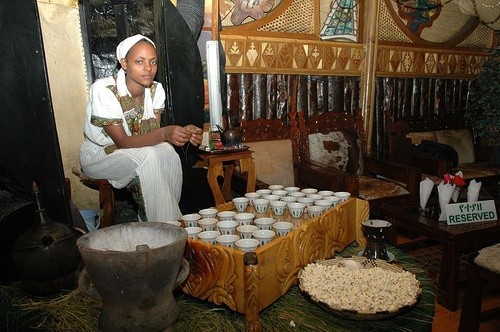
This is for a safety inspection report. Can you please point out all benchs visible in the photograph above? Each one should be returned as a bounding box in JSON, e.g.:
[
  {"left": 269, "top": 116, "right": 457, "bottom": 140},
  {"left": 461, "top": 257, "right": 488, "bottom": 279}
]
[{"left": 384, "top": 108, "right": 500, "bottom": 196}]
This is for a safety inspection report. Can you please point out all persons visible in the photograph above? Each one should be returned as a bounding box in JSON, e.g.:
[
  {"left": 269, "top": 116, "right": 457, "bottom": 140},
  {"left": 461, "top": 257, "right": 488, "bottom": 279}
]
[{"left": 80, "top": 35, "right": 202, "bottom": 227}]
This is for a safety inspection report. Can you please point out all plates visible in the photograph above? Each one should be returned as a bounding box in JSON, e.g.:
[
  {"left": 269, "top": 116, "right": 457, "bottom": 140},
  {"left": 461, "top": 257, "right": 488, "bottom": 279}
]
[{"left": 357, "top": 250, "right": 396, "bottom": 263}]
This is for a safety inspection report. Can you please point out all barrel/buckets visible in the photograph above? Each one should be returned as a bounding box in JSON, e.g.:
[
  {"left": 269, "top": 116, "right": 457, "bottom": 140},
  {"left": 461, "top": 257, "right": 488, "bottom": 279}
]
[{"left": 79, "top": 209, "right": 97, "bottom": 232}]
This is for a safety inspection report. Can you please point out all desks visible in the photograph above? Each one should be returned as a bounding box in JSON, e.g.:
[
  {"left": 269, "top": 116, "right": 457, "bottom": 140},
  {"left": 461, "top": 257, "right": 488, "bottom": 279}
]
[
  {"left": 0, "top": 239, "right": 435, "bottom": 332},
  {"left": 197, "top": 151, "right": 258, "bottom": 206},
  {"left": 381, "top": 199, "right": 500, "bottom": 311}
]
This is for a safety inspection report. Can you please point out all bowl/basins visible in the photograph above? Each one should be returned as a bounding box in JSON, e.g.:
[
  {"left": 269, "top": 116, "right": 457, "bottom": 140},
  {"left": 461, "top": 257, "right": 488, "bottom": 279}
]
[
  {"left": 231, "top": 184, "right": 351, "bottom": 219},
  {"left": 165, "top": 207, "right": 293, "bottom": 251}
]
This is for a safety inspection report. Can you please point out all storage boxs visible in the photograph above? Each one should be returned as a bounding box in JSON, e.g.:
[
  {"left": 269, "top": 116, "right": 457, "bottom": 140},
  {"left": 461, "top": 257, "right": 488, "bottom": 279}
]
[{"left": 179, "top": 184, "right": 356, "bottom": 332}]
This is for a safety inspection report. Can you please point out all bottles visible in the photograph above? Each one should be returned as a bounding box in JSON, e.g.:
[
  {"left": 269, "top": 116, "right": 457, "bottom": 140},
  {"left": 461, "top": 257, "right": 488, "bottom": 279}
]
[{"left": 361, "top": 219, "right": 392, "bottom": 261}]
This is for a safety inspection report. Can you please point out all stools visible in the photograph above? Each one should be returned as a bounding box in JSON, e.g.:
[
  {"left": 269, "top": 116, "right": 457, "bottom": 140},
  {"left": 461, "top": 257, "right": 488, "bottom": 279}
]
[{"left": 72, "top": 166, "right": 120, "bottom": 227}]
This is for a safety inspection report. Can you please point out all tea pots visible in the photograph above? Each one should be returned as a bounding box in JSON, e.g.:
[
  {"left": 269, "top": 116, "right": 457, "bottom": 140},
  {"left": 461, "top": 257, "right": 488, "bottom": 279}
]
[{"left": 216, "top": 118, "right": 246, "bottom": 146}]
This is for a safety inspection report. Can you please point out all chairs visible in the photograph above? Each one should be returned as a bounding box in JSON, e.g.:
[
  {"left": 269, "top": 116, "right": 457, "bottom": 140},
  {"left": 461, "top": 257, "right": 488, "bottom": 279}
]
[
  {"left": 230, "top": 114, "right": 343, "bottom": 199},
  {"left": 298, "top": 107, "right": 421, "bottom": 219},
  {"left": 458, "top": 242, "right": 500, "bottom": 332}
]
